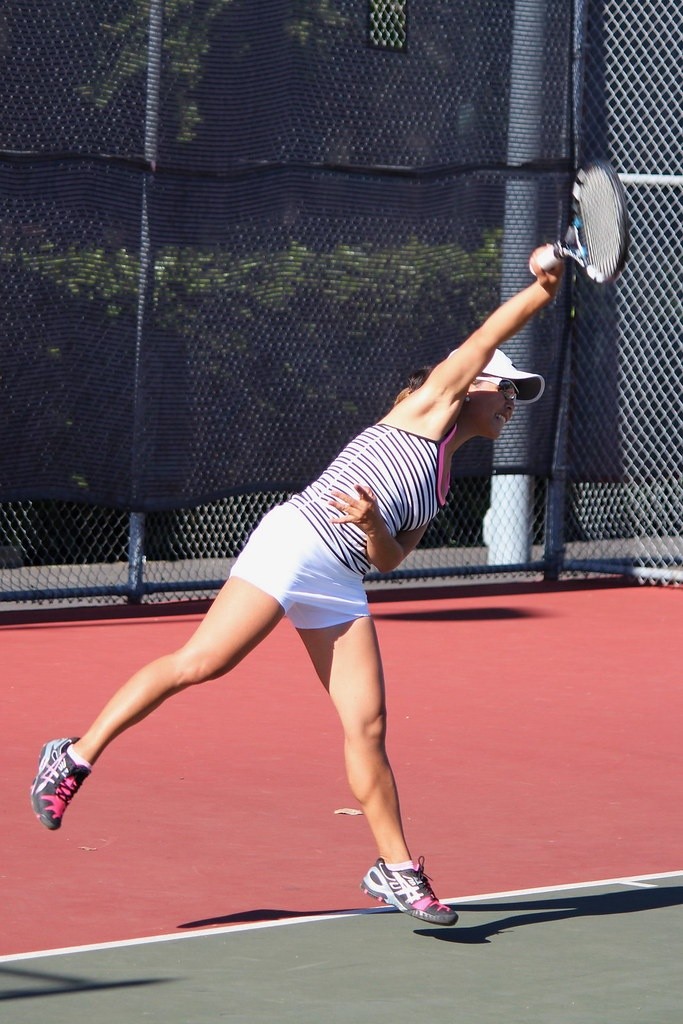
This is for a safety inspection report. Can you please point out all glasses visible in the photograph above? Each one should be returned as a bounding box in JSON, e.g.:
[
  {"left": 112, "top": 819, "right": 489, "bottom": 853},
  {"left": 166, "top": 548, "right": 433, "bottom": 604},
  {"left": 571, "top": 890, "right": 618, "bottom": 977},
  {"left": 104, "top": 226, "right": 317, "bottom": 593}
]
[{"left": 476, "top": 376, "right": 519, "bottom": 405}]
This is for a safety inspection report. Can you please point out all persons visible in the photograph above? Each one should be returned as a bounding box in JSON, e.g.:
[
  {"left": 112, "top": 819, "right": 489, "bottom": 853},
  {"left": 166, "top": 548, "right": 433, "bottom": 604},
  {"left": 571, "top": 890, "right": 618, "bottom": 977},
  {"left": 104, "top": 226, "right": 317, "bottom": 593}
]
[{"left": 29, "top": 242, "right": 563, "bottom": 926}]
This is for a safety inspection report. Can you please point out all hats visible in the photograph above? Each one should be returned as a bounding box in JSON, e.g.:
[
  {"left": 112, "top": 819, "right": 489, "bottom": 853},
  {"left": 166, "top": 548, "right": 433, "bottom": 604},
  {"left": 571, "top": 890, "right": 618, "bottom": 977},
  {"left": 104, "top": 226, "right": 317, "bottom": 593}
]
[{"left": 482, "top": 349, "right": 545, "bottom": 405}]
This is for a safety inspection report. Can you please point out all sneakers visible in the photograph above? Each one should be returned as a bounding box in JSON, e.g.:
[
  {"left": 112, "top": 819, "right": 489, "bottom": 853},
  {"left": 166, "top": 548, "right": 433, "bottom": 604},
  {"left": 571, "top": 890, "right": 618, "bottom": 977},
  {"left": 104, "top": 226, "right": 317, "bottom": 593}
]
[
  {"left": 30, "top": 737, "right": 91, "bottom": 830},
  {"left": 360, "top": 856, "right": 459, "bottom": 925}
]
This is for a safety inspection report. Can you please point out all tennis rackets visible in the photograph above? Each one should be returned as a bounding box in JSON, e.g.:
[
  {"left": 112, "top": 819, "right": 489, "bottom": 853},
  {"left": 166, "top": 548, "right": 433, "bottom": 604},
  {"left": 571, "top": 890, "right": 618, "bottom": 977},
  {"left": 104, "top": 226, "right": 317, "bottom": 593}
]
[{"left": 531, "top": 158, "right": 629, "bottom": 286}]
